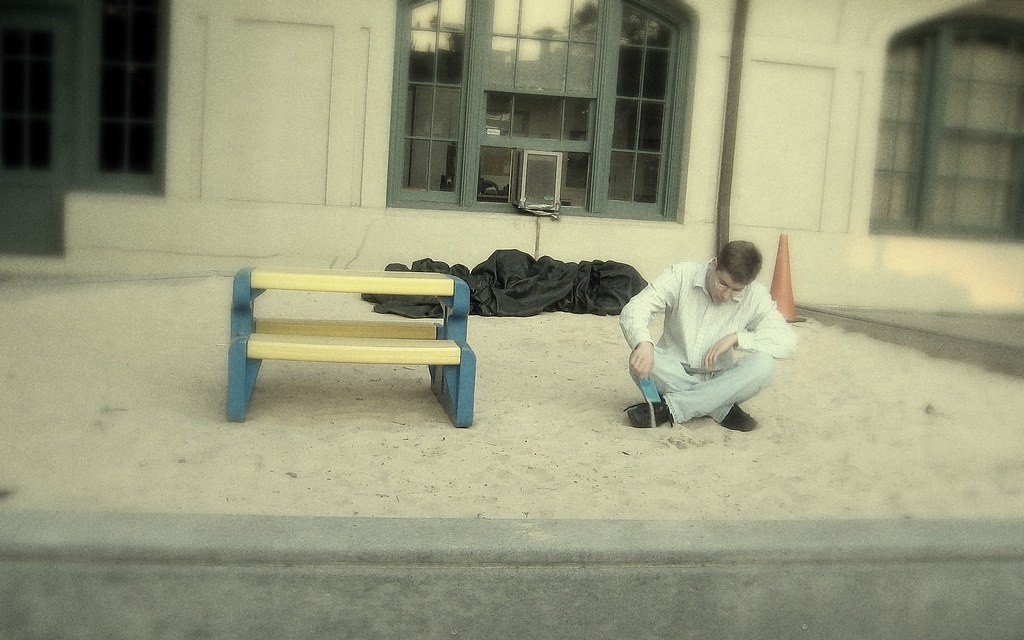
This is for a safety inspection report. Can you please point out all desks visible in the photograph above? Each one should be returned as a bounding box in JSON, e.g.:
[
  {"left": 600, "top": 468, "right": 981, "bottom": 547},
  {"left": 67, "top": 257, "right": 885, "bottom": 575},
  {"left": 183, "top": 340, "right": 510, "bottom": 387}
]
[{"left": 231, "top": 265, "right": 470, "bottom": 342}]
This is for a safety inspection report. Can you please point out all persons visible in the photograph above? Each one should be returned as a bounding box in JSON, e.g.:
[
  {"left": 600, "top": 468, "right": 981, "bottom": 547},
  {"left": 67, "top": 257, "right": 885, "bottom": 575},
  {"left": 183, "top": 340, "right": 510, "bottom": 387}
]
[{"left": 619, "top": 241, "right": 796, "bottom": 431}]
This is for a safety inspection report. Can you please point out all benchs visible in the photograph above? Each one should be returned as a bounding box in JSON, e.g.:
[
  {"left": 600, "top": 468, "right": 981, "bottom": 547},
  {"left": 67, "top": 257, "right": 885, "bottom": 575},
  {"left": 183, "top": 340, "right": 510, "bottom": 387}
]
[{"left": 226, "top": 321, "right": 477, "bottom": 428}]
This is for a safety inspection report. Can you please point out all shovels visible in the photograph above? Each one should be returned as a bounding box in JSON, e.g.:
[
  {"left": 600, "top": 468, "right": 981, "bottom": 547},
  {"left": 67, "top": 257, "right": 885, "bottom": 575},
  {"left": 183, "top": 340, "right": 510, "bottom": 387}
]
[{"left": 630, "top": 372, "right": 662, "bottom": 403}]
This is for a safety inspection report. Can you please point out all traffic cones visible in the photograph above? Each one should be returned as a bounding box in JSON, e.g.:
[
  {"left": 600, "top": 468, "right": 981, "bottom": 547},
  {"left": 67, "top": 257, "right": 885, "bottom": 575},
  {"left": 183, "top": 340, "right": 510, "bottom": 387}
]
[{"left": 770, "top": 234, "right": 808, "bottom": 323}]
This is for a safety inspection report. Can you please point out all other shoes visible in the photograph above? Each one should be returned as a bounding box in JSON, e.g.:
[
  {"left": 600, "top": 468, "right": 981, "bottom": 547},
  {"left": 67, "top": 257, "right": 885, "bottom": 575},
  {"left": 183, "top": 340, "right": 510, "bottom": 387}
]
[
  {"left": 719, "top": 403, "right": 758, "bottom": 432},
  {"left": 623, "top": 396, "right": 674, "bottom": 428}
]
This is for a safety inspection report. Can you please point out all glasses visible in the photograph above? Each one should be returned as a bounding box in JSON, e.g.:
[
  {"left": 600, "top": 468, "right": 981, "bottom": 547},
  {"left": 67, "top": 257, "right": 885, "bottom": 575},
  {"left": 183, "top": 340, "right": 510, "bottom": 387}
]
[{"left": 714, "top": 269, "right": 749, "bottom": 302}]
